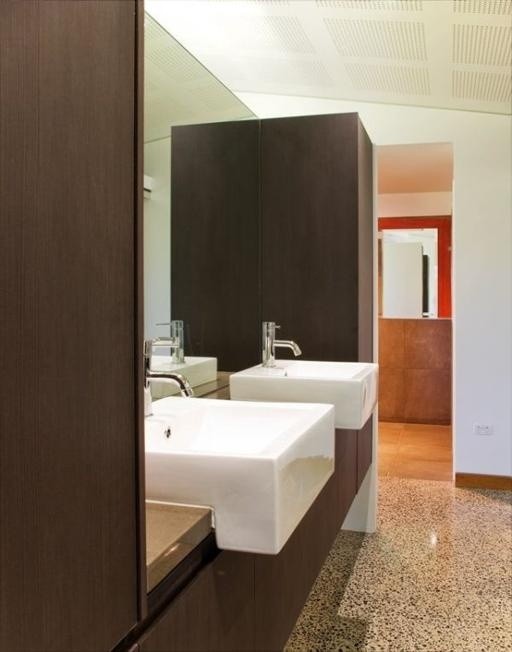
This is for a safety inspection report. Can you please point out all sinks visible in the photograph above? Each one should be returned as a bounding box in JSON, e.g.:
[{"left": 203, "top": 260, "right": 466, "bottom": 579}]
[
  {"left": 150, "top": 355, "right": 218, "bottom": 388},
  {"left": 144, "top": 395, "right": 336, "bottom": 556},
  {"left": 229, "top": 359, "right": 379, "bottom": 430}
]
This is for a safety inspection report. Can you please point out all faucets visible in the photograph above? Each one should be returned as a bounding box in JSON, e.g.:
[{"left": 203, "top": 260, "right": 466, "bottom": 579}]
[
  {"left": 261, "top": 322, "right": 302, "bottom": 368},
  {"left": 143, "top": 340, "right": 195, "bottom": 418},
  {"left": 150, "top": 320, "right": 184, "bottom": 364}
]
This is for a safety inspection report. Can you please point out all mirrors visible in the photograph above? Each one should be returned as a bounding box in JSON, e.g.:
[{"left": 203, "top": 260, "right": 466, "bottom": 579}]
[{"left": 140, "top": 10, "right": 262, "bottom": 420}]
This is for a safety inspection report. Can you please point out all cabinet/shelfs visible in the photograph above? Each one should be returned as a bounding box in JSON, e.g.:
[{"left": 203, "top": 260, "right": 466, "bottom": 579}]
[{"left": 77, "top": 414, "right": 377, "bottom": 651}]
